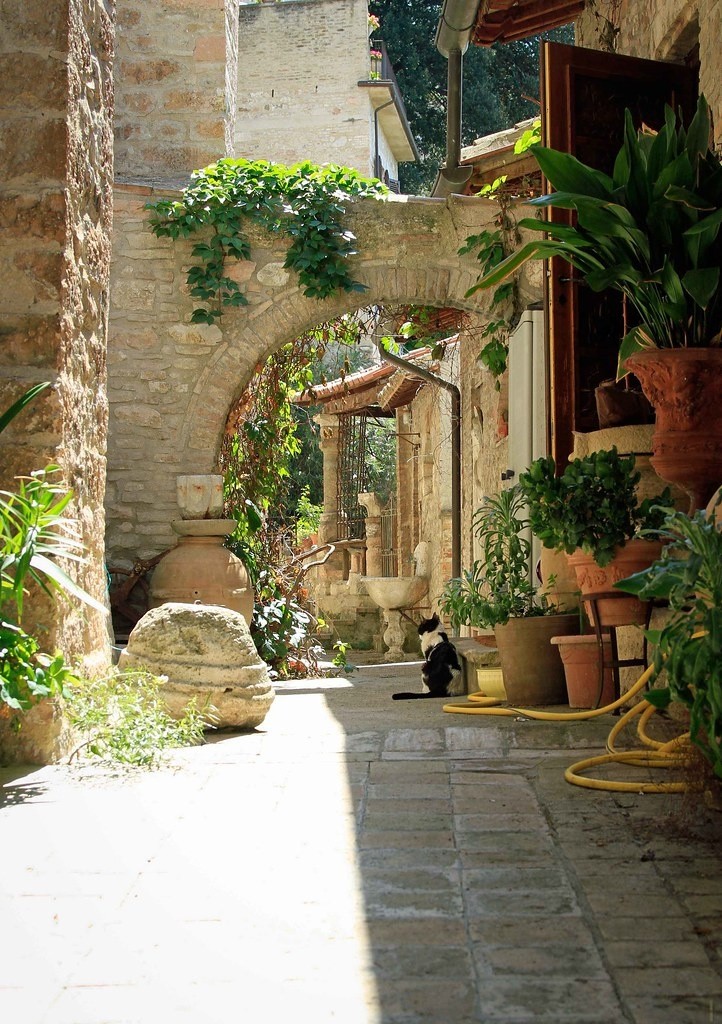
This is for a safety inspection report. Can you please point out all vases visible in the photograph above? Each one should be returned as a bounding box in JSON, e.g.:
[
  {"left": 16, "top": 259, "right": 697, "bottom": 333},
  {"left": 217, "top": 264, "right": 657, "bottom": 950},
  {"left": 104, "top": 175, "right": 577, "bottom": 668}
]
[
  {"left": 367, "top": 25, "right": 374, "bottom": 37},
  {"left": 371, "top": 60, "right": 381, "bottom": 72},
  {"left": 475, "top": 667, "right": 507, "bottom": 701},
  {"left": 550, "top": 634, "right": 616, "bottom": 708}
]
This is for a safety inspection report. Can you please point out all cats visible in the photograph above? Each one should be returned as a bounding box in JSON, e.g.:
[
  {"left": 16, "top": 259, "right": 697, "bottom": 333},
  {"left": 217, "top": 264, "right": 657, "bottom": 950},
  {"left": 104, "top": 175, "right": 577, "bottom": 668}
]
[{"left": 392, "top": 613, "right": 463, "bottom": 701}]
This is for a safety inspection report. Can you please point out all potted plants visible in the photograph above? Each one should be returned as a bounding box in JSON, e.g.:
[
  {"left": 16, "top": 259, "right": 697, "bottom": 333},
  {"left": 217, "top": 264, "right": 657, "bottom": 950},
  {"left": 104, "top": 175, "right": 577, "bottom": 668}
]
[
  {"left": 519, "top": 445, "right": 675, "bottom": 627},
  {"left": 432, "top": 482, "right": 580, "bottom": 704},
  {"left": 296, "top": 485, "right": 323, "bottom": 552},
  {"left": 464, "top": 86, "right": 721, "bottom": 522}
]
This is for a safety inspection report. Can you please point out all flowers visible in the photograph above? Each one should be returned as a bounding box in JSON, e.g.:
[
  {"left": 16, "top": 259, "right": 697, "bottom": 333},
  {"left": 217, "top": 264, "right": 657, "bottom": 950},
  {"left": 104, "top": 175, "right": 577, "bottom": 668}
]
[
  {"left": 368, "top": 13, "right": 381, "bottom": 31},
  {"left": 370, "top": 50, "right": 383, "bottom": 61}
]
